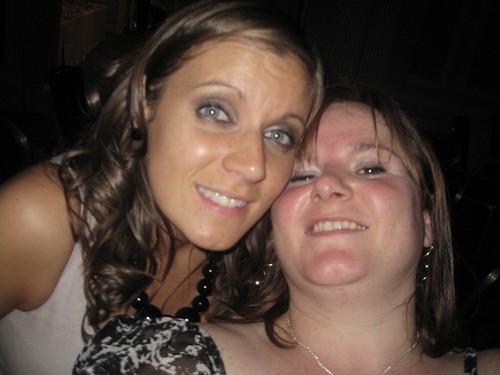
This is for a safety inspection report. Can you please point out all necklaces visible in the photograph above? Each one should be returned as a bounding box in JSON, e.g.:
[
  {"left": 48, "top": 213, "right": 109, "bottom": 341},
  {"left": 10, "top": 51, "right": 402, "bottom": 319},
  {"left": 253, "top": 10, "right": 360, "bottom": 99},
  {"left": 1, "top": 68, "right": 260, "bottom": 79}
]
[
  {"left": 286, "top": 310, "right": 421, "bottom": 375},
  {"left": 127, "top": 244, "right": 221, "bottom": 323}
]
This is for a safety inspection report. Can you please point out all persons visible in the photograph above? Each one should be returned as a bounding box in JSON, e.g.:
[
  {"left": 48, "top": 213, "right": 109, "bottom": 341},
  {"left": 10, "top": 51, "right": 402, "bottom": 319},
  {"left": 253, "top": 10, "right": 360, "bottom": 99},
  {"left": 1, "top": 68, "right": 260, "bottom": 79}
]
[
  {"left": 0, "top": 0, "right": 328, "bottom": 375},
  {"left": 69, "top": 79, "right": 500, "bottom": 375}
]
